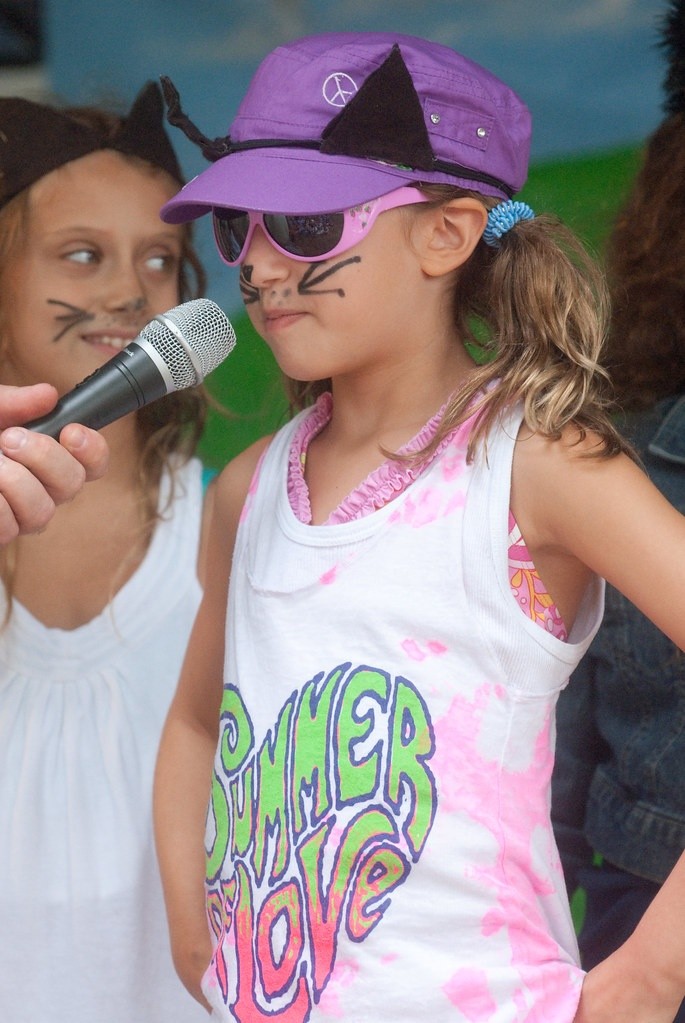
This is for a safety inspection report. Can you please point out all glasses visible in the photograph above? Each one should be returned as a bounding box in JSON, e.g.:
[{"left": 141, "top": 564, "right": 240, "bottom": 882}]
[{"left": 212, "top": 187, "right": 457, "bottom": 267}]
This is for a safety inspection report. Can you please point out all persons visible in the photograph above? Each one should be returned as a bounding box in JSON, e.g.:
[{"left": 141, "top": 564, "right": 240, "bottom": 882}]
[
  {"left": 0, "top": 80, "right": 217, "bottom": 1023},
  {"left": 0, "top": 381, "right": 111, "bottom": 548},
  {"left": 554, "top": 1, "right": 685, "bottom": 1023},
  {"left": 151, "top": 35, "right": 684, "bottom": 1023}
]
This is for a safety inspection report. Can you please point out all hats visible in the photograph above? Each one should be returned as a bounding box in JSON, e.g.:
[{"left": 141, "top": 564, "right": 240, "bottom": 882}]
[{"left": 159, "top": 29, "right": 532, "bottom": 224}]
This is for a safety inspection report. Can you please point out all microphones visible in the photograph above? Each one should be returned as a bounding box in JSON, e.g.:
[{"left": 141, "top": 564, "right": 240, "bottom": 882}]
[{"left": 20, "top": 298, "right": 238, "bottom": 444}]
